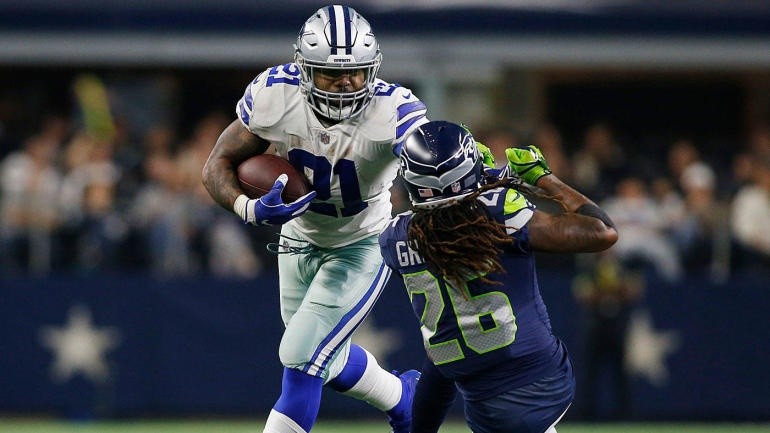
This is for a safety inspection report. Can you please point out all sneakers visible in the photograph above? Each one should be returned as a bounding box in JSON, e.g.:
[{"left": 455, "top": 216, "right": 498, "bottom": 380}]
[{"left": 386, "top": 369, "right": 421, "bottom": 433}]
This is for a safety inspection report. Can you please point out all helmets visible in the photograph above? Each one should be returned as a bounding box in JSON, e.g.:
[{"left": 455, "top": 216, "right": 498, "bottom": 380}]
[
  {"left": 401, "top": 122, "right": 480, "bottom": 210},
  {"left": 293, "top": 4, "right": 383, "bottom": 121}
]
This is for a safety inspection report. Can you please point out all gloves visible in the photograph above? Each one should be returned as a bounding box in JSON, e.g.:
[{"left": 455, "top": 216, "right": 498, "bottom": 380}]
[
  {"left": 233, "top": 173, "right": 316, "bottom": 227},
  {"left": 475, "top": 142, "right": 495, "bottom": 169},
  {"left": 506, "top": 145, "right": 552, "bottom": 186}
]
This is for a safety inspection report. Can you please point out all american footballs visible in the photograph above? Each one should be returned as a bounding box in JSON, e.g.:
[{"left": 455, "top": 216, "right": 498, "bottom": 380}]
[{"left": 235, "top": 154, "right": 307, "bottom": 204}]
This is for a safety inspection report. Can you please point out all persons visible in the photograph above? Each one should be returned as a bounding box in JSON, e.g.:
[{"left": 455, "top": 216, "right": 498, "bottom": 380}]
[
  {"left": 0, "top": 113, "right": 263, "bottom": 287},
  {"left": 378, "top": 120, "right": 620, "bottom": 433},
  {"left": 201, "top": 5, "right": 493, "bottom": 433},
  {"left": 491, "top": 120, "right": 770, "bottom": 279}
]
